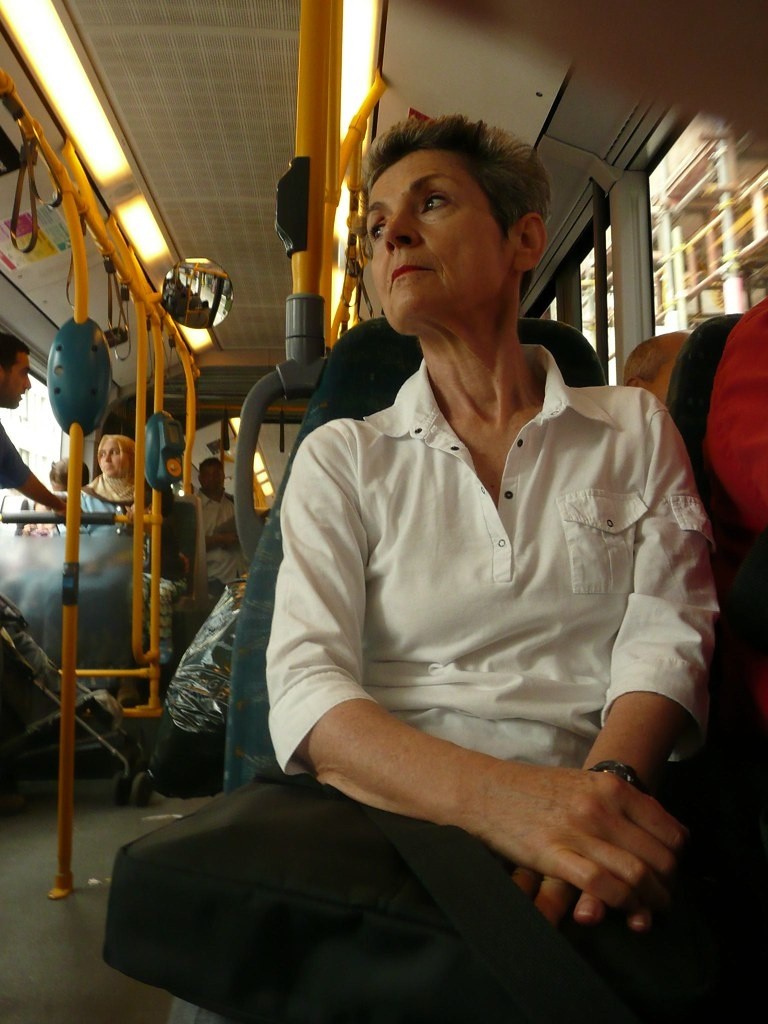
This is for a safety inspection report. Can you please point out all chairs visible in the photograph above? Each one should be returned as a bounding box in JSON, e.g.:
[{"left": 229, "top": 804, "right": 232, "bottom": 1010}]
[
  {"left": 166, "top": 495, "right": 209, "bottom": 652},
  {"left": 226, "top": 316, "right": 601, "bottom": 797},
  {"left": 668, "top": 315, "right": 768, "bottom": 701},
  {"left": 0, "top": 492, "right": 68, "bottom": 535}
]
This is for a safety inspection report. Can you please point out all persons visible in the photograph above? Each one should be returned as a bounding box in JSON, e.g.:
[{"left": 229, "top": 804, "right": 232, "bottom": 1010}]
[
  {"left": 23, "top": 413, "right": 191, "bottom": 708},
  {"left": 0, "top": 332, "right": 84, "bottom": 517},
  {"left": 705, "top": 295, "right": 768, "bottom": 732},
  {"left": 192, "top": 457, "right": 243, "bottom": 595},
  {"left": 166, "top": 116, "right": 721, "bottom": 1023},
  {"left": 623, "top": 331, "right": 688, "bottom": 403}
]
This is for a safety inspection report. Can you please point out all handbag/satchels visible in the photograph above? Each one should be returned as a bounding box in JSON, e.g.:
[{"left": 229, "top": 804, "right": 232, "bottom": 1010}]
[
  {"left": 146, "top": 572, "right": 247, "bottom": 799},
  {"left": 103, "top": 775, "right": 724, "bottom": 1024}
]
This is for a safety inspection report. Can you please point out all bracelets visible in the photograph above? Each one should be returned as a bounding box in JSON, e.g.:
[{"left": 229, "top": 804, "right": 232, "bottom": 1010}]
[{"left": 585, "top": 760, "right": 649, "bottom": 795}]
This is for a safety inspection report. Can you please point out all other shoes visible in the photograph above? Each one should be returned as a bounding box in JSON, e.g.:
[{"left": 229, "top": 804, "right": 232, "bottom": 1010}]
[{"left": 0, "top": 793, "right": 25, "bottom": 816}]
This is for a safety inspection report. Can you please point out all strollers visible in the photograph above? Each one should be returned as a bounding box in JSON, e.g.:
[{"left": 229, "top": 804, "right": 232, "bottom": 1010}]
[{"left": 0, "top": 593, "right": 154, "bottom": 806}]
